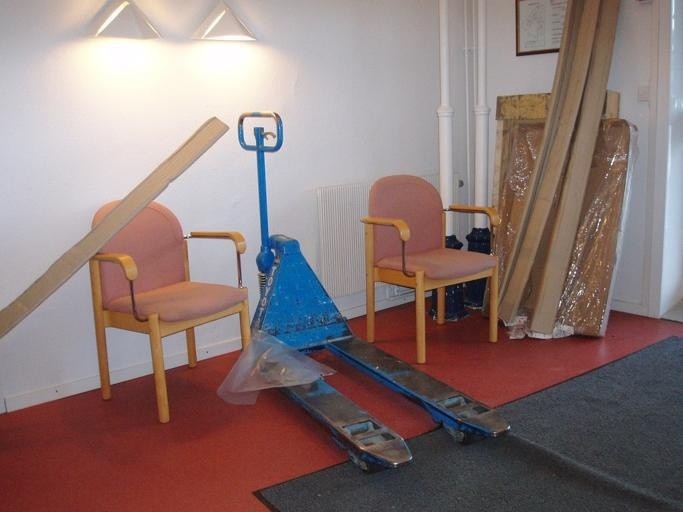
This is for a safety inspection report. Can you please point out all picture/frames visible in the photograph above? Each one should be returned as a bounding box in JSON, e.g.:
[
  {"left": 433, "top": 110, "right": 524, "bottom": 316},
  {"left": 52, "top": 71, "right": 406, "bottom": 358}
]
[{"left": 515, "top": 0, "right": 568, "bottom": 58}]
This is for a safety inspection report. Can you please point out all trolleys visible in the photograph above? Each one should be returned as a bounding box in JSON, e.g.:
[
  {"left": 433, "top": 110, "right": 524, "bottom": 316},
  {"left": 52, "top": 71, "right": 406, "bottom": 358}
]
[{"left": 238, "top": 112, "right": 510, "bottom": 472}]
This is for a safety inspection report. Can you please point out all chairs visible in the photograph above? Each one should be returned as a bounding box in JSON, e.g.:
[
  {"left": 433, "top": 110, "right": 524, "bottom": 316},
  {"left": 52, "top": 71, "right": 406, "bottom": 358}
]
[
  {"left": 363, "top": 173, "right": 500, "bottom": 365},
  {"left": 89, "top": 201, "right": 251, "bottom": 426}
]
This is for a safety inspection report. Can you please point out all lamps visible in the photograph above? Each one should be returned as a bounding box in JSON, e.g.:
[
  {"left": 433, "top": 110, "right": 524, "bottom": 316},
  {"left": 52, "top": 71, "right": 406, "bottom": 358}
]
[
  {"left": 95, "top": 0, "right": 161, "bottom": 41},
  {"left": 191, "top": 0, "right": 254, "bottom": 41}
]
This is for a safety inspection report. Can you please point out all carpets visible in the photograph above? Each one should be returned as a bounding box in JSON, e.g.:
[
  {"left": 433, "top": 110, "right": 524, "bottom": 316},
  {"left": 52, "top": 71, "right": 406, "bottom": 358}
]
[{"left": 249, "top": 334, "right": 682, "bottom": 512}]
